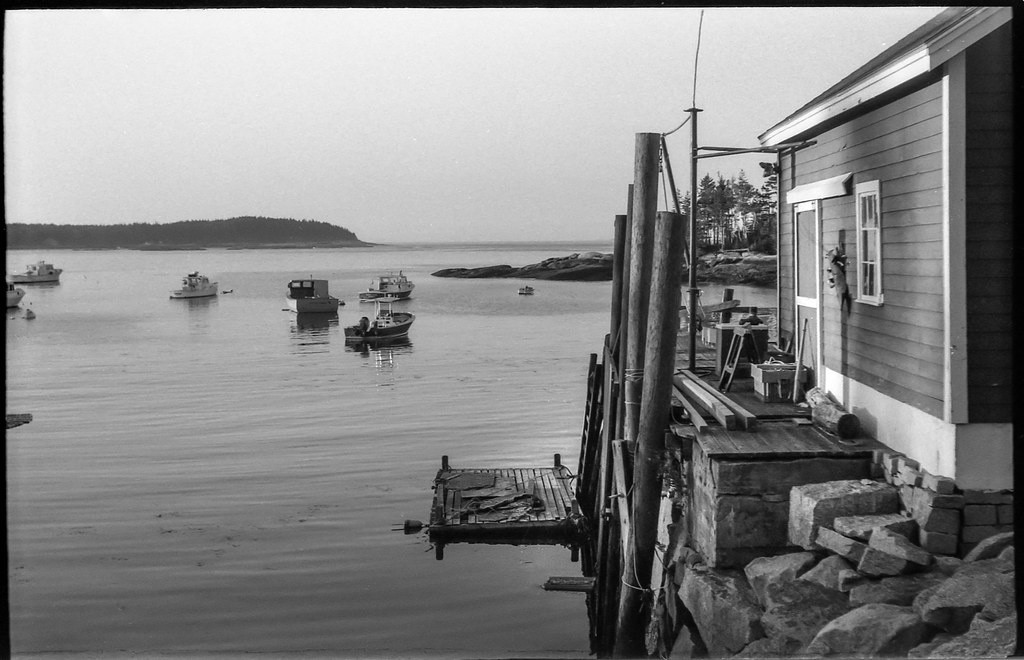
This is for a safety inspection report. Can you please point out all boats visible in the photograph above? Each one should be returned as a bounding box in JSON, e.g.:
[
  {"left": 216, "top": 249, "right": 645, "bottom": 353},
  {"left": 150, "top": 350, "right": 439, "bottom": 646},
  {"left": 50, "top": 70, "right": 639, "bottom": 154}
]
[
  {"left": 359, "top": 270, "right": 415, "bottom": 300},
  {"left": 169, "top": 271, "right": 218, "bottom": 297},
  {"left": 285, "top": 279, "right": 339, "bottom": 313},
  {"left": 344, "top": 296, "right": 415, "bottom": 339},
  {"left": 6, "top": 279, "right": 25, "bottom": 306},
  {"left": 518, "top": 285, "right": 534, "bottom": 295},
  {"left": 8, "top": 260, "right": 63, "bottom": 283}
]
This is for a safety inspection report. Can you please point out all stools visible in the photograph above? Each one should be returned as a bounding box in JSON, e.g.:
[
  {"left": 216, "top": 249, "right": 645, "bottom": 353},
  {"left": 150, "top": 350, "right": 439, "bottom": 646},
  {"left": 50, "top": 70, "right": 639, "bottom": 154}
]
[{"left": 715, "top": 323, "right": 769, "bottom": 378}]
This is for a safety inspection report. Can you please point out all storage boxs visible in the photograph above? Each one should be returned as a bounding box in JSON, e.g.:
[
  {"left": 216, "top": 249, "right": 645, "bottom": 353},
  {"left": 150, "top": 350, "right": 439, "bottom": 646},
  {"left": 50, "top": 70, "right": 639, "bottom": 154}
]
[{"left": 749, "top": 363, "right": 807, "bottom": 404}]
